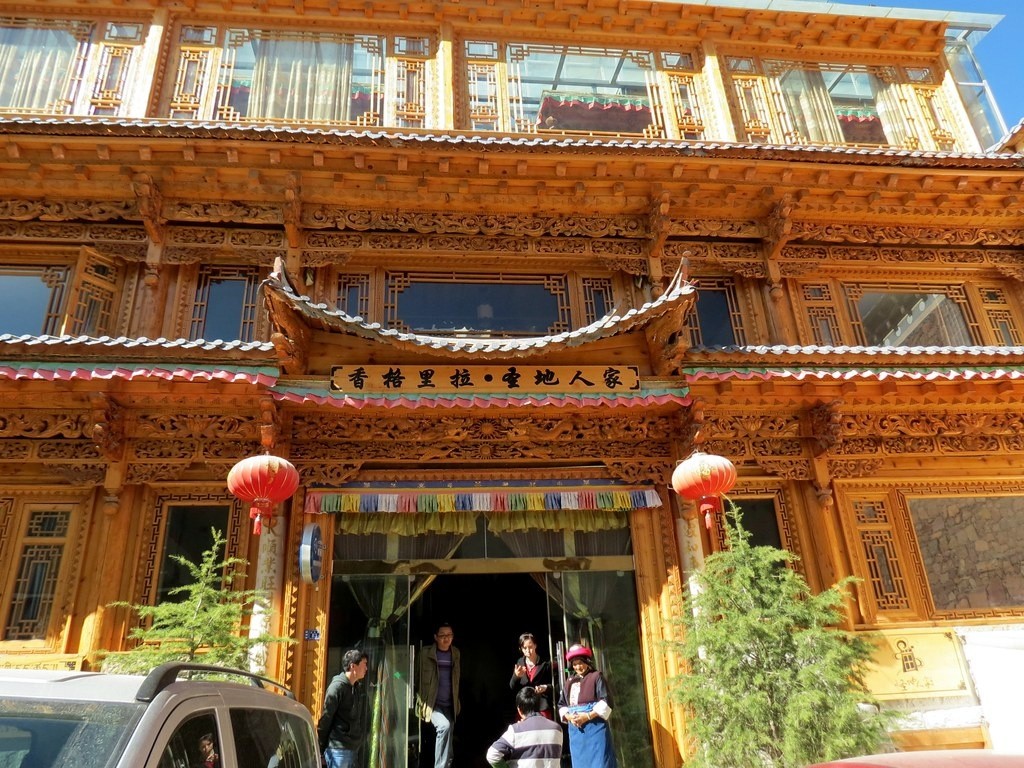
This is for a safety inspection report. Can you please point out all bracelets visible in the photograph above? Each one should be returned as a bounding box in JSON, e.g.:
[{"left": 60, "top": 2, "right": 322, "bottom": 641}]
[{"left": 587, "top": 712, "right": 591, "bottom": 720}]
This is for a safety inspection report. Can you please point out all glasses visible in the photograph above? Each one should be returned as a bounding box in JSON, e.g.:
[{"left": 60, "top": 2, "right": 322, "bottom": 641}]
[{"left": 437, "top": 633, "right": 453, "bottom": 639}]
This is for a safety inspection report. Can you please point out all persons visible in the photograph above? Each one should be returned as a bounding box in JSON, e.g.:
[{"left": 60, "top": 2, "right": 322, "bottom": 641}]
[
  {"left": 316, "top": 652, "right": 372, "bottom": 768},
  {"left": 485, "top": 632, "right": 619, "bottom": 768},
  {"left": 196, "top": 733, "right": 222, "bottom": 768},
  {"left": 268, "top": 741, "right": 298, "bottom": 768},
  {"left": 413, "top": 622, "right": 461, "bottom": 768}
]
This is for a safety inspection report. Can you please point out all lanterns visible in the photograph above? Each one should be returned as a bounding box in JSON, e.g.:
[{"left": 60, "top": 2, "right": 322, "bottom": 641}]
[
  {"left": 227, "top": 453, "right": 300, "bottom": 535},
  {"left": 671, "top": 452, "right": 737, "bottom": 529}
]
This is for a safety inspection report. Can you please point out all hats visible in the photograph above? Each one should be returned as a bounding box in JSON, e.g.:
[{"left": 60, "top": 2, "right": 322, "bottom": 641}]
[{"left": 565, "top": 644, "right": 591, "bottom": 661}]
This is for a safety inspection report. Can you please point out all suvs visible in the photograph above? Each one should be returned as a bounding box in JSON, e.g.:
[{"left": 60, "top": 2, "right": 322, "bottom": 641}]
[{"left": 0, "top": 661, "right": 321, "bottom": 768}]
